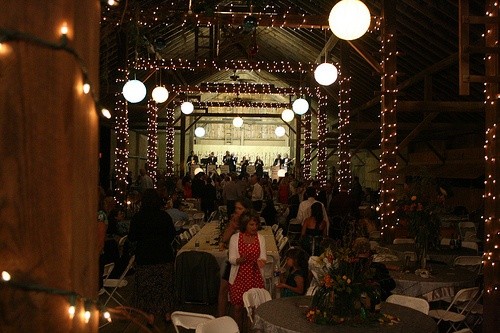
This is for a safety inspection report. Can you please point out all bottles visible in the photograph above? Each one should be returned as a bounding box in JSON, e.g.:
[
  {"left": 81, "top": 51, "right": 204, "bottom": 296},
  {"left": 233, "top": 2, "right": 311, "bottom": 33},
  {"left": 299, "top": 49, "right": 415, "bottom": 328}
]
[
  {"left": 273, "top": 264, "right": 280, "bottom": 285},
  {"left": 220, "top": 219, "right": 225, "bottom": 230}
]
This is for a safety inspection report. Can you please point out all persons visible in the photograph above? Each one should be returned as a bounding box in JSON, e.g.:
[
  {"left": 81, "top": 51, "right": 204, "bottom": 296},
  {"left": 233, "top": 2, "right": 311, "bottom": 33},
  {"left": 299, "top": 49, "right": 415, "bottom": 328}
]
[
  {"left": 218, "top": 197, "right": 308, "bottom": 333},
  {"left": 99, "top": 188, "right": 178, "bottom": 333},
  {"left": 127, "top": 169, "right": 242, "bottom": 222},
  {"left": 240, "top": 156, "right": 249, "bottom": 171},
  {"left": 186, "top": 150, "right": 199, "bottom": 172},
  {"left": 207, "top": 151, "right": 217, "bottom": 165},
  {"left": 273, "top": 153, "right": 294, "bottom": 172},
  {"left": 325, "top": 176, "right": 380, "bottom": 243},
  {"left": 254, "top": 155, "right": 263, "bottom": 178},
  {"left": 240, "top": 171, "right": 329, "bottom": 272},
  {"left": 223, "top": 150, "right": 238, "bottom": 173},
  {"left": 396, "top": 200, "right": 442, "bottom": 267},
  {"left": 337, "top": 237, "right": 396, "bottom": 302}
]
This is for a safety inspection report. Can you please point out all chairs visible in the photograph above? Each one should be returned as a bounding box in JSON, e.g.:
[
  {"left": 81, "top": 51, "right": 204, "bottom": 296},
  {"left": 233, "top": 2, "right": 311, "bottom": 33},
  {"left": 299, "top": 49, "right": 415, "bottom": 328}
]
[{"left": 99, "top": 187, "right": 485, "bottom": 333}]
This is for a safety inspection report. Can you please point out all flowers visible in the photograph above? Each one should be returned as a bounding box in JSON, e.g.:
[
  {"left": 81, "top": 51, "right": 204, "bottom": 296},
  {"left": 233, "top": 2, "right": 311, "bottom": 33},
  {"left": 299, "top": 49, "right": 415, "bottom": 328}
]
[
  {"left": 394, "top": 185, "right": 450, "bottom": 265},
  {"left": 306, "top": 223, "right": 400, "bottom": 326}
]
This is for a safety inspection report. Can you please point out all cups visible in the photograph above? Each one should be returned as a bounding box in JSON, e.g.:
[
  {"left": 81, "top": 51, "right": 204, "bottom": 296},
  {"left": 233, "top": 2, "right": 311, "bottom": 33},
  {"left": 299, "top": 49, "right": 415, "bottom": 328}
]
[
  {"left": 195, "top": 240, "right": 198, "bottom": 248},
  {"left": 206, "top": 236, "right": 210, "bottom": 244}
]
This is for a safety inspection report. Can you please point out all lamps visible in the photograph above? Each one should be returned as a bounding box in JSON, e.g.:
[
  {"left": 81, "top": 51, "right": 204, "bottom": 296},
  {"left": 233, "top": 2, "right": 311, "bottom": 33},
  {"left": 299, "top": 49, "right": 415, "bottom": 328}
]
[
  {"left": 275, "top": 125, "right": 285, "bottom": 137},
  {"left": 181, "top": 97, "right": 194, "bottom": 114},
  {"left": 314, "top": 29, "right": 338, "bottom": 86},
  {"left": 233, "top": 116, "right": 243, "bottom": 128},
  {"left": 329, "top": 0, "right": 371, "bottom": 41},
  {"left": 123, "top": 73, "right": 147, "bottom": 103},
  {"left": 292, "top": 70, "right": 309, "bottom": 115},
  {"left": 195, "top": 127, "right": 205, "bottom": 138},
  {"left": 151, "top": 68, "right": 169, "bottom": 103},
  {"left": 282, "top": 108, "right": 295, "bottom": 122}
]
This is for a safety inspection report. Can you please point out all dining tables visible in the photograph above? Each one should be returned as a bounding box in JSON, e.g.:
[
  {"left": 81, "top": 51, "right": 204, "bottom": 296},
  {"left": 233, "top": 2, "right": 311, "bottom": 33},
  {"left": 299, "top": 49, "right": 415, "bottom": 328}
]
[
  {"left": 388, "top": 269, "right": 473, "bottom": 302},
  {"left": 251, "top": 295, "right": 437, "bottom": 333},
  {"left": 175, "top": 220, "right": 281, "bottom": 273}
]
